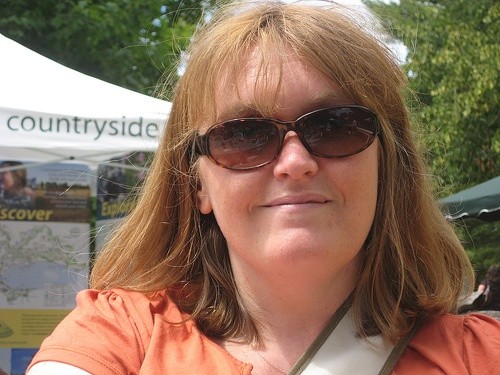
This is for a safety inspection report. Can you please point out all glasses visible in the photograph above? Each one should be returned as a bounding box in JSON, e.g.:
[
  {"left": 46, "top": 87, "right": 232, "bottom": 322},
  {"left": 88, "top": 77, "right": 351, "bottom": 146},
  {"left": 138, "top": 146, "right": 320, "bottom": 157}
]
[{"left": 176, "top": 98, "right": 383, "bottom": 165}]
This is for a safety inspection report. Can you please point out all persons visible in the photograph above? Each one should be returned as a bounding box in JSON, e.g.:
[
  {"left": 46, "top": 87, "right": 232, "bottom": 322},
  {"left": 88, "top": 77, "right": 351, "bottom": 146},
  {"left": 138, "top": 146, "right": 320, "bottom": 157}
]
[
  {"left": 477, "top": 264, "right": 499, "bottom": 310},
  {"left": 25, "top": 0, "right": 500, "bottom": 375}
]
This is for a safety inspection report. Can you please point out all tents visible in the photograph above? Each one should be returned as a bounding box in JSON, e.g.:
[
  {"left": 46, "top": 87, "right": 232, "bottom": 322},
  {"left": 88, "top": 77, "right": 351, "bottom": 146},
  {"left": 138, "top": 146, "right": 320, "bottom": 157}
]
[
  {"left": 0, "top": 31, "right": 175, "bottom": 160},
  {"left": 436, "top": 174, "right": 499, "bottom": 221}
]
